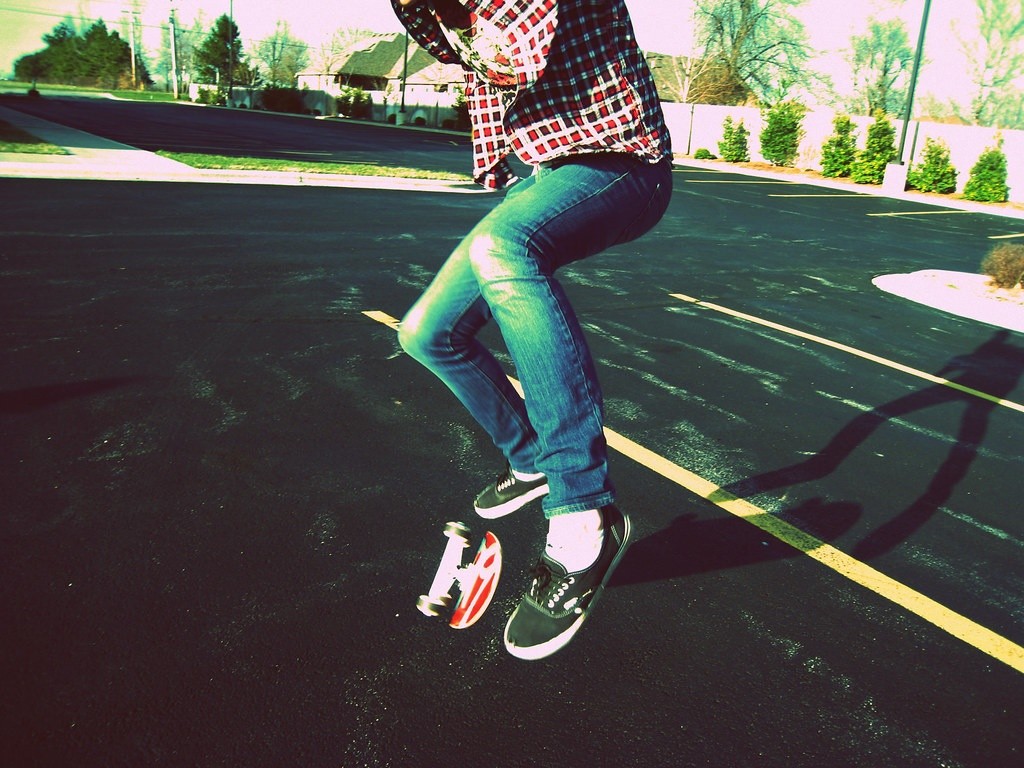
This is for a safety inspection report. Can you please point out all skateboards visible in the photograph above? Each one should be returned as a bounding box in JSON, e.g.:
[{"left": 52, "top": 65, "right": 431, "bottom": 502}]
[{"left": 416, "top": 520, "right": 502, "bottom": 630}]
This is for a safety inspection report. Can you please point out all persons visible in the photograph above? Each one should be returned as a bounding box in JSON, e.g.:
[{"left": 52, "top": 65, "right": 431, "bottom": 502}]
[{"left": 390, "top": 0, "right": 674, "bottom": 661}]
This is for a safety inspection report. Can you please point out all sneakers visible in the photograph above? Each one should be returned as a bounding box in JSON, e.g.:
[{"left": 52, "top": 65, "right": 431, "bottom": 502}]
[
  {"left": 503, "top": 502, "right": 633, "bottom": 658},
  {"left": 473, "top": 467, "right": 549, "bottom": 519}
]
